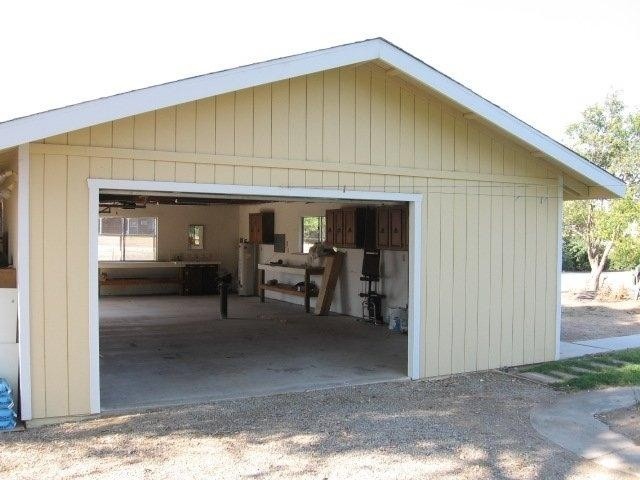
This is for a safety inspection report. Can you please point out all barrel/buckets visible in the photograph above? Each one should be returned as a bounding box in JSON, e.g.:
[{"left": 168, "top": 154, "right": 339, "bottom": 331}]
[{"left": 387, "top": 307, "right": 408, "bottom": 333}]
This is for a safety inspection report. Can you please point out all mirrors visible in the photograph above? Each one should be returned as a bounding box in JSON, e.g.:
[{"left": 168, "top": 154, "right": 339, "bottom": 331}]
[{"left": 188, "top": 224, "right": 205, "bottom": 249}]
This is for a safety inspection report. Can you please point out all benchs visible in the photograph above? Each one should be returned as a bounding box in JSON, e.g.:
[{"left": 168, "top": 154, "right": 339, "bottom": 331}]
[{"left": 99, "top": 266, "right": 182, "bottom": 296}]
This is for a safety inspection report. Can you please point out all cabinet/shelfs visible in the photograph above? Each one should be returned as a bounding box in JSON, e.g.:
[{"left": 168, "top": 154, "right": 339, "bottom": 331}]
[
  {"left": 376, "top": 205, "right": 409, "bottom": 251},
  {"left": 184, "top": 264, "right": 218, "bottom": 296},
  {"left": 324, "top": 207, "right": 366, "bottom": 249},
  {"left": 247, "top": 211, "right": 274, "bottom": 244},
  {"left": 258, "top": 262, "right": 325, "bottom": 313}
]
